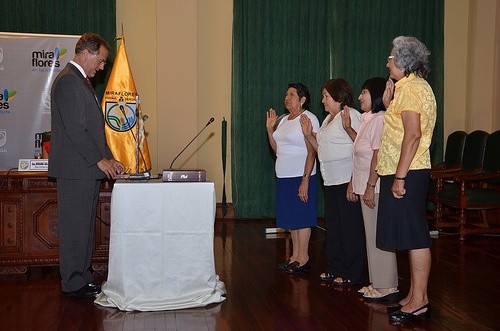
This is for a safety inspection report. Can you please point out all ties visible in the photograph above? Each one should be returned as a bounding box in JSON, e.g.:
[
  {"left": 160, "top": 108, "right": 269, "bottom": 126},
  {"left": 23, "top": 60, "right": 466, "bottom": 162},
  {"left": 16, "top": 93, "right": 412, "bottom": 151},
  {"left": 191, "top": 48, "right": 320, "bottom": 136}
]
[{"left": 85, "top": 77, "right": 96, "bottom": 98}]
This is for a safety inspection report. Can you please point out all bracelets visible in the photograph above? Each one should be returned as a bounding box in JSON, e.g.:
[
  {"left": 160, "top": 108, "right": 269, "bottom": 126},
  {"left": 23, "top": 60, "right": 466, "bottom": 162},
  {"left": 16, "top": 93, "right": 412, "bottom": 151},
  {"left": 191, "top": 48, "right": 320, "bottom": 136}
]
[
  {"left": 304, "top": 174, "right": 310, "bottom": 178},
  {"left": 395, "top": 177, "right": 405, "bottom": 180},
  {"left": 367, "top": 183, "right": 375, "bottom": 187}
]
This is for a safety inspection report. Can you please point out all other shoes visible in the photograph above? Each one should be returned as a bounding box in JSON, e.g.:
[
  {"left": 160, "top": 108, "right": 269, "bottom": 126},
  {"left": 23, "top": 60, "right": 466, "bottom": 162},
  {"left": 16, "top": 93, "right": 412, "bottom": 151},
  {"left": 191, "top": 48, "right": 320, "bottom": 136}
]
[
  {"left": 285, "top": 258, "right": 312, "bottom": 273},
  {"left": 388, "top": 303, "right": 433, "bottom": 321},
  {"left": 387, "top": 303, "right": 403, "bottom": 310},
  {"left": 275, "top": 258, "right": 291, "bottom": 270}
]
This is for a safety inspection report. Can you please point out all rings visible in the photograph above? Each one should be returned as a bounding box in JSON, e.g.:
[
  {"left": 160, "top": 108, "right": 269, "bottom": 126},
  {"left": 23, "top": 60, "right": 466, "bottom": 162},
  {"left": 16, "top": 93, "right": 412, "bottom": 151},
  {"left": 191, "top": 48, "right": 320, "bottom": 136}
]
[{"left": 301, "top": 123, "right": 304, "bottom": 126}]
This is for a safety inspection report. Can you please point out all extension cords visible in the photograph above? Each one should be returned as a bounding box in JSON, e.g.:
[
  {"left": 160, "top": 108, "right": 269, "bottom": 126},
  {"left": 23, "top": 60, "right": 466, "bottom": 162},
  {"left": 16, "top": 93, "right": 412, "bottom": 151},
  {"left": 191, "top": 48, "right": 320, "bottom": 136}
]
[
  {"left": 429, "top": 230, "right": 439, "bottom": 235},
  {"left": 266, "top": 228, "right": 286, "bottom": 233}
]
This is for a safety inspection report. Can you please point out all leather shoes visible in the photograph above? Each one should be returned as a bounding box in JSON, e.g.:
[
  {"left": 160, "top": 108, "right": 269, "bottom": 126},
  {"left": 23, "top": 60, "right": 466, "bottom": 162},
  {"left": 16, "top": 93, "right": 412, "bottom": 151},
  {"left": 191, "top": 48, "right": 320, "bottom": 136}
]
[{"left": 61, "top": 283, "right": 102, "bottom": 298}]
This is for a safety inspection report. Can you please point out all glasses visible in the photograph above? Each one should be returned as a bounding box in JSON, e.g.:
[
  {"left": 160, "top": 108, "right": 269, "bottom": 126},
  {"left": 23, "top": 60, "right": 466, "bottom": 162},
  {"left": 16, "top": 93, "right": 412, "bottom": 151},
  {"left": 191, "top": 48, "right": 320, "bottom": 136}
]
[
  {"left": 85, "top": 47, "right": 107, "bottom": 67},
  {"left": 387, "top": 55, "right": 394, "bottom": 62}
]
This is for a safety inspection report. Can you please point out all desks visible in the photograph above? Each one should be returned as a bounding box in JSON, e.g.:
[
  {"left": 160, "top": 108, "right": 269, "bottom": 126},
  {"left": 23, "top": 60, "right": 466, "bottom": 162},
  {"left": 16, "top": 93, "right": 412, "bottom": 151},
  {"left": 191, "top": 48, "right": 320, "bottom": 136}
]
[
  {"left": 0, "top": 170, "right": 116, "bottom": 276},
  {"left": 94, "top": 177, "right": 227, "bottom": 312}
]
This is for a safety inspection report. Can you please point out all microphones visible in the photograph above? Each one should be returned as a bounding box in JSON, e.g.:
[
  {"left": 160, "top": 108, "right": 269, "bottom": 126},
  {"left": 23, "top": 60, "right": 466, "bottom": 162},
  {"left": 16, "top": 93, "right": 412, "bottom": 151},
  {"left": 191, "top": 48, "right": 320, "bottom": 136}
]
[
  {"left": 169, "top": 117, "right": 214, "bottom": 169},
  {"left": 119, "top": 105, "right": 151, "bottom": 179}
]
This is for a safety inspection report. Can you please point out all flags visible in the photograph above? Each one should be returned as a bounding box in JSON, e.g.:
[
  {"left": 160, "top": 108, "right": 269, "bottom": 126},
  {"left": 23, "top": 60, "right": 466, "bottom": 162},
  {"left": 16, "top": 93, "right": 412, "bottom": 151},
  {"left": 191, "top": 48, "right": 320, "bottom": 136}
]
[{"left": 101, "top": 39, "right": 152, "bottom": 173}]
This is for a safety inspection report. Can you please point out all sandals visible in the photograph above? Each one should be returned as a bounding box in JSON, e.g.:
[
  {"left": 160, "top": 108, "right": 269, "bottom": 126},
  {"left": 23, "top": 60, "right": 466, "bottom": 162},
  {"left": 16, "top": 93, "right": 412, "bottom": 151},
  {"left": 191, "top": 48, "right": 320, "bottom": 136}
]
[
  {"left": 321, "top": 269, "right": 341, "bottom": 281},
  {"left": 332, "top": 275, "right": 367, "bottom": 284},
  {"left": 358, "top": 284, "right": 374, "bottom": 293},
  {"left": 363, "top": 286, "right": 400, "bottom": 297}
]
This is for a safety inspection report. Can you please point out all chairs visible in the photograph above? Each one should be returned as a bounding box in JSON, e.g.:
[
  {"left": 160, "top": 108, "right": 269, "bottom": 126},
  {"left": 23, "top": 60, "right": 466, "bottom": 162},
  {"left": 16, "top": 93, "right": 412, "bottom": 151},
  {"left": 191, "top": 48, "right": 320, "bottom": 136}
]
[{"left": 426, "top": 130, "right": 500, "bottom": 240}]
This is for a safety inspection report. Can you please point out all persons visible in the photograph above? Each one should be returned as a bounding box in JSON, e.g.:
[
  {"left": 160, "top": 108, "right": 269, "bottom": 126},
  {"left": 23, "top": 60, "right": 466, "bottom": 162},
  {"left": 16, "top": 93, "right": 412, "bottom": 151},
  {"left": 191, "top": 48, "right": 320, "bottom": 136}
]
[
  {"left": 266, "top": 84, "right": 319, "bottom": 274},
  {"left": 341, "top": 78, "right": 400, "bottom": 300},
  {"left": 300, "top": 78, "right": 366, "bottom": 285},
  {"left": 48, "top": 33, "right": 125, "bottom": 296},
  {"left": 376, "top": 36, "right": 437, "bottom": 322}
]
